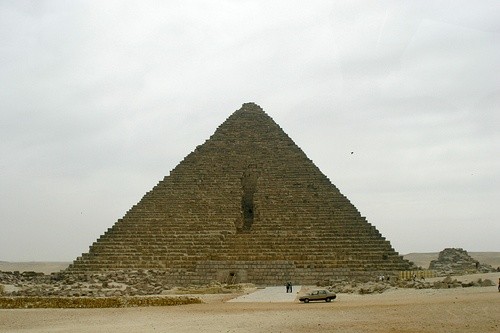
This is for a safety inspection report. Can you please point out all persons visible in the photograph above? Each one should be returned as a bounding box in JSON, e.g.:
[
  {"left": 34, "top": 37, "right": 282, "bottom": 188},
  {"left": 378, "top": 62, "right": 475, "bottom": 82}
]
[
  {"left": 286, "top": 281, "right": 290, "bottom": 293},
  {"left": 289, "top": 282, "right": 293, "bottom": 294}
]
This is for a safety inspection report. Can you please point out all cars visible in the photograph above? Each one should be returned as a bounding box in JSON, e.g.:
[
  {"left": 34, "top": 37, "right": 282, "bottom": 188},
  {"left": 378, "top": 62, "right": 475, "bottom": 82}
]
[{"left": 299, "top": 289, "right": 336, "bottom": 303}]
